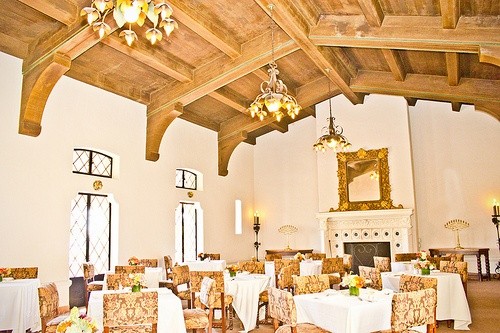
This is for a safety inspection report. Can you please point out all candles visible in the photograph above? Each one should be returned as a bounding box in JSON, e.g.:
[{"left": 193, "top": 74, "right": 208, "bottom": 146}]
[
  {"left": 493, "top": 204, "right": 500, "bottom": 215},
  {"left": 253, "top": 211, "right": 260, "bottom": 224}
]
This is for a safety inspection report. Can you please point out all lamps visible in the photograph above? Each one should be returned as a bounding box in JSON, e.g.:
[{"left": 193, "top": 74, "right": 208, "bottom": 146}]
[
  {"left": 80, "top": 0, "right": 177, "bottom": 45},
  {"left": 312, "top": 70, "right": 352, "bottom": 153},
  {"left": 246, "top": 4, "right": 301, "bottom": 123}
]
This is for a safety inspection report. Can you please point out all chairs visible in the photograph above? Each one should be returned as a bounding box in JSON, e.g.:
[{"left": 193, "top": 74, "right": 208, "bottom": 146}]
[{"left": 0, "top": 252, "right": 470, "bottom": 333}]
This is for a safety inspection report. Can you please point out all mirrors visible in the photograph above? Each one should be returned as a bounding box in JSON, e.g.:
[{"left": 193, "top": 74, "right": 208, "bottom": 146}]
[{"left": 334, "top": 149, "right": 395, "bottom": 209}]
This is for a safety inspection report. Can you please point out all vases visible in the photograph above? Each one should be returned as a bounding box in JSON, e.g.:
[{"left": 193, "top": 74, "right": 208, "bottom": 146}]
[
  {"left": 421, "top": 267, "right": 430, "bottom": 275},
  {"left": 421, "top": 258, "right": 425, "bottom": 261},
  {"left": 348, "top": 285, "right": 359, "bottom": 296},
  {"left": 131, "top": 284, "right": 140, "bottom": 292},
  {"left": 230, "top": 271, "right": 237, "bottom": 279}
]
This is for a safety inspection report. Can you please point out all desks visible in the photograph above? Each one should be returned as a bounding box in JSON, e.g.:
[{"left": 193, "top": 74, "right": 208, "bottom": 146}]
[
  {"left": 391, "top": 261, "right": 418, "bottom": 272},
  {"left": 102, "top": 267, "right": 162, "bottom": 291},
  {"left": 299, "top": 261, "right": 326, "bottom": 276},
  {"left": 381, "top": 271, "right": 473, "bottom": 331},
  {"left": 0, "top": 277, "right": 42, "bottom": 332},
  {"left": 426, "top": 248, "right": 491, "bottom": 282},
  {"left": 266, "top": 250, "right": 313, "bottom": 257},
  {"left": 201, "top": 272, "right": 268, "bottom": 332},
  {"left": 87, "top": 288, "right": 186, "bottom": 333},
  {"left": 181, "top": 259, "right": 227, "bottom": 273},
  {"left": 292, "top": 288, "right": 428, "bottom": 333}
]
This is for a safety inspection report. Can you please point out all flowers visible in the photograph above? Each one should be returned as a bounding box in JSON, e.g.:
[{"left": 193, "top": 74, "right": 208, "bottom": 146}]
[
  {"left": 55, "top": 306, "right": 98, "bottom": 333},
  {"left": 228, "top": 264, "right": 239, "bottom": 272},
  {"left": 420, "top": 249, "right": 427, "bottom": 259},
  {"left": 128, "top": 256, "right": 140, "bottom": 265},
  {"left": 129, "top": 272, "right": 142, "bottom": 285},
  {"left": 415, "top": 261, "right": 434, "bottom": 270},
  {"left": 339, "top": 271, "right": 365, "bottom": 289}
]
